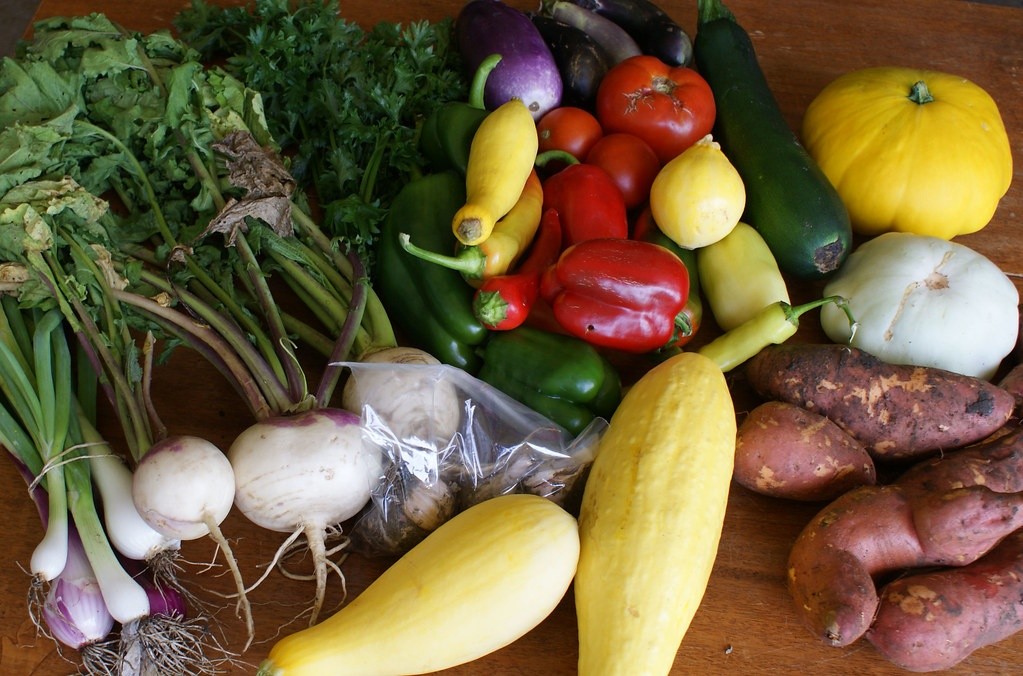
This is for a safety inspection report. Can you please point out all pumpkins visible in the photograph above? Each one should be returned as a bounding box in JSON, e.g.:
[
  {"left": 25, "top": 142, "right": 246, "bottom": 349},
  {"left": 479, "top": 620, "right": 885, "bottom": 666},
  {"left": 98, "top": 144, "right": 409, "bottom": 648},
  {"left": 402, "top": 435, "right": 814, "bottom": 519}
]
[{"left": 797, "top": 63, "right": 1020, "bottom": 381}]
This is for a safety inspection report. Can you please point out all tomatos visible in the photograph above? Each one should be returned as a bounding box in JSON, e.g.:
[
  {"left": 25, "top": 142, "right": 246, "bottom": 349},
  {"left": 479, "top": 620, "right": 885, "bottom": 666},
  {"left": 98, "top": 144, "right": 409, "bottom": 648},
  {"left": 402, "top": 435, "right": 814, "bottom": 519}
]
[
  {"left": 594, "top": 56, "right": 717, "bottom": 160},
  {"left": 537, "top": 106, "right": 603, "bottom": 163},
  {"left": 584, "top": 132, "right": 660, "bottom": 210}
]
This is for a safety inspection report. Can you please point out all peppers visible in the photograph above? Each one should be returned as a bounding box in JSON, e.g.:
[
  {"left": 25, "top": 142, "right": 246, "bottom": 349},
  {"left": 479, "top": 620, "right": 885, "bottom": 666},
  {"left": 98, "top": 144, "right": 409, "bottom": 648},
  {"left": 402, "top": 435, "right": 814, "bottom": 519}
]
[{"left": 368, "top": 54, "right": 859, "bottom": 440}]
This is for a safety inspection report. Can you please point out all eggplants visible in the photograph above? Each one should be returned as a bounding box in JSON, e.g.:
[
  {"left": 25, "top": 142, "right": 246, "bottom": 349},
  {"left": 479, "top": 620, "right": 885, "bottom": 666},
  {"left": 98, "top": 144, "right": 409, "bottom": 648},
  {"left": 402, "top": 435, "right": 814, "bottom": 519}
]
[{"left": 449, "top": 0, "right": 691, "bottom": 120}]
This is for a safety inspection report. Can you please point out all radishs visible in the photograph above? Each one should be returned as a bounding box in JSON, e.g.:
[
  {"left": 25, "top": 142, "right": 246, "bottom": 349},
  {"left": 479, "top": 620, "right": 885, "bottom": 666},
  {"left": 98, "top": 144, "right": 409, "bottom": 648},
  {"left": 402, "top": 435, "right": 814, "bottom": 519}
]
[{"left": 129, "top": 348, "right": 460, "bottom": 628}]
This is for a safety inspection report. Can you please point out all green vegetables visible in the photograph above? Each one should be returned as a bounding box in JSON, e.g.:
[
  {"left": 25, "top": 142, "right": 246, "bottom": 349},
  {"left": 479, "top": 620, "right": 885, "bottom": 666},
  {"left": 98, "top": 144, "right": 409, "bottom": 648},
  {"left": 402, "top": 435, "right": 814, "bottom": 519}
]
[{"left": 166, "top": 0, "right": 474, "bottom": 287}]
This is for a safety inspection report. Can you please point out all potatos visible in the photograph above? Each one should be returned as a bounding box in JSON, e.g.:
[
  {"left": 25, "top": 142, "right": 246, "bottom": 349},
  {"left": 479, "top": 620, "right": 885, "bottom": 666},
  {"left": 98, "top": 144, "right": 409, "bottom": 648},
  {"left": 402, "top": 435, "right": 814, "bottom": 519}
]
[{"left": 733, "top": 341, "right": 1023, "bottom": 673}]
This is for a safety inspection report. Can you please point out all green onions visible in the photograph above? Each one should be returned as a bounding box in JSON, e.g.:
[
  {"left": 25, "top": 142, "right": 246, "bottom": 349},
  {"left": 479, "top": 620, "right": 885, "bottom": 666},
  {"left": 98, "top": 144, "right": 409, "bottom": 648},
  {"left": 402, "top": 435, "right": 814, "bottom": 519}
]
[{"left": 0, "top": 297, "right": 240, "bottom": 676}]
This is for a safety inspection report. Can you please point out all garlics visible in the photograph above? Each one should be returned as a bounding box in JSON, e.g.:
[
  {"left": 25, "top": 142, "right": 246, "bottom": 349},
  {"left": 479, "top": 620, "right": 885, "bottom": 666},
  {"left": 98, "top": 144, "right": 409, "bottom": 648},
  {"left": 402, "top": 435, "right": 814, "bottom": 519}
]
[{"left": 359, "top": 443, "right": 597, "bottom": 557}]
